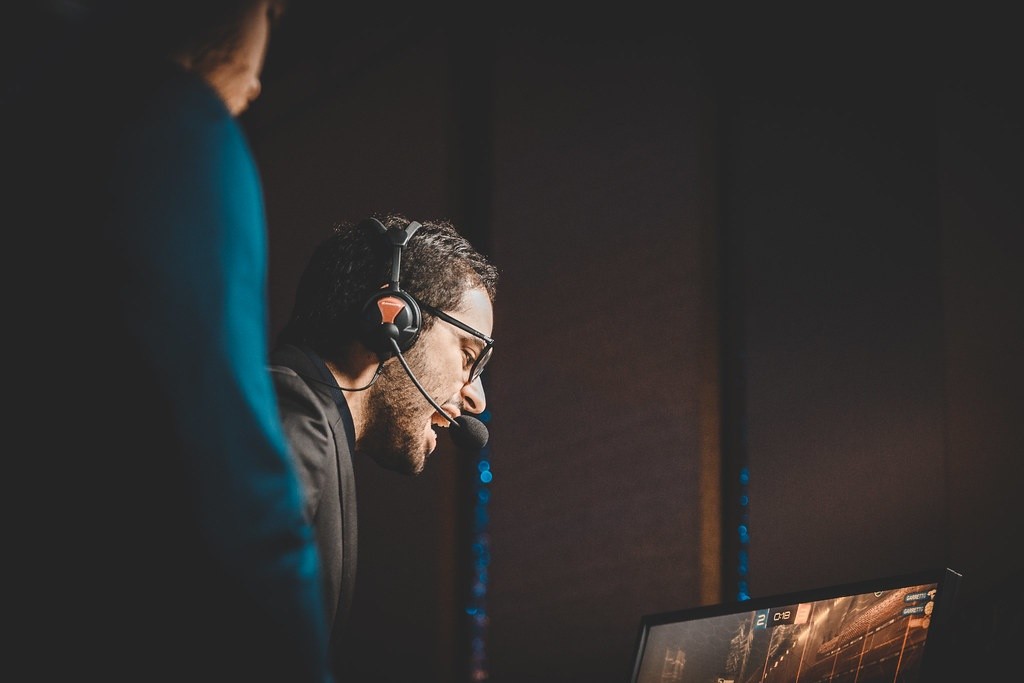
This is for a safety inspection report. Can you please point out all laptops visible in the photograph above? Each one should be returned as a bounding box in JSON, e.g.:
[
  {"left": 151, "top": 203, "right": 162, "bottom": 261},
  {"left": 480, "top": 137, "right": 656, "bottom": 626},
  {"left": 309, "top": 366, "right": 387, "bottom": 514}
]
[{"left": 629, "top": 567, "right": 963, "bottom": 683}]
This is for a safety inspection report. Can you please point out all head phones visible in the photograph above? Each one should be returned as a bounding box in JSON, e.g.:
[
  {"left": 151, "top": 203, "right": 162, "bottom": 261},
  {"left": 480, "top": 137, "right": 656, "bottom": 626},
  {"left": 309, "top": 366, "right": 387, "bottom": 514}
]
[{"left": 362, "top": 221, "right": 422, "bottom": 359}]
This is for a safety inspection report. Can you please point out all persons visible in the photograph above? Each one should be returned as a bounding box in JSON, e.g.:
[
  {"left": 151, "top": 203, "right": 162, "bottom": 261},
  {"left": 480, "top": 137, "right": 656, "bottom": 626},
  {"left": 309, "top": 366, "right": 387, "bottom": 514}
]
[
  {"left": 264, "top": 214, "right": 497, "bottom": 660},
  {"left": 0, "top": 0, "right": 328, "bottom": 681}
]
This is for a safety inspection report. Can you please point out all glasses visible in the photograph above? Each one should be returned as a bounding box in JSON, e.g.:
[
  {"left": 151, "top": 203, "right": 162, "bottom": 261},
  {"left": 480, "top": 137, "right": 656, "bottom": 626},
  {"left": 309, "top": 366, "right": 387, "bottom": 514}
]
[{"left": 418, "top": 299, "right": 497, "bottom": 385}]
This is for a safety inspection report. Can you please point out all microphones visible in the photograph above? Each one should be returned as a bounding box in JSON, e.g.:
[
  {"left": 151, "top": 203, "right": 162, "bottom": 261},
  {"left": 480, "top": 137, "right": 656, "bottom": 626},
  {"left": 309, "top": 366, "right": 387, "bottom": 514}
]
[{"left": 378, "top": 322, "right": 489, "bottom": 450}]
